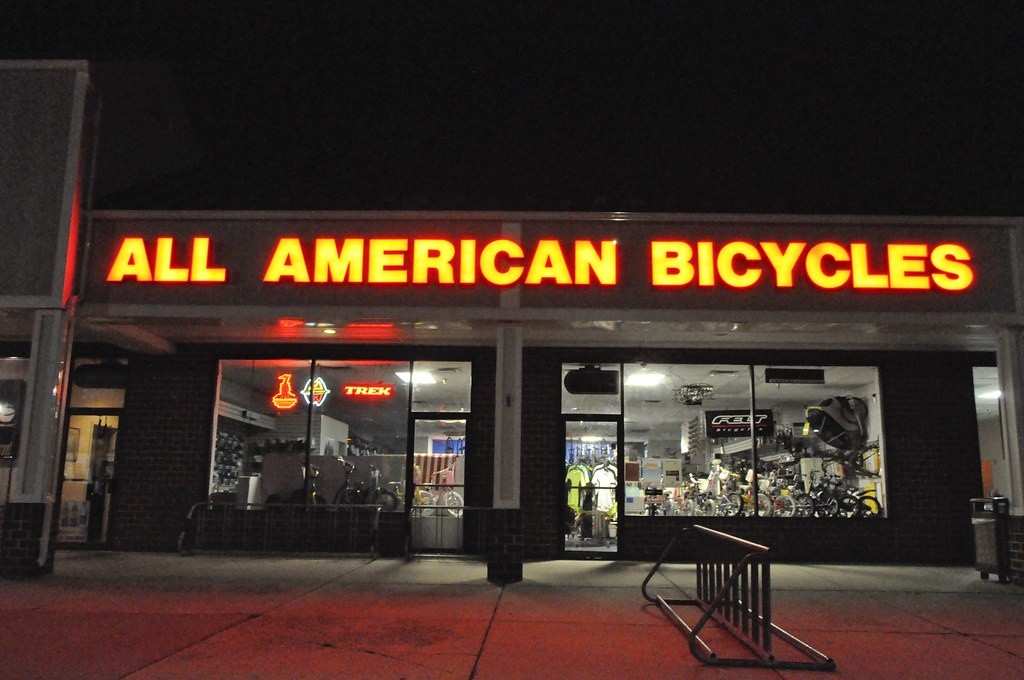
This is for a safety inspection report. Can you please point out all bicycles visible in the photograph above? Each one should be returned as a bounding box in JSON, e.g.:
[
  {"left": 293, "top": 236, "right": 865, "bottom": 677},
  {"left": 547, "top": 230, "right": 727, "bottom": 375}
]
[
  {"left": 640, "top": 458, "right": 882, "bottom": 520},
  {"left": 569, "top": 438, "right": 615, "bottom": 467},
  {"left": 267, "top": 455, "right": 464, "bottom": 516},
  {"left": 444, "top": 436, "right": 466, "bottom": 454}
]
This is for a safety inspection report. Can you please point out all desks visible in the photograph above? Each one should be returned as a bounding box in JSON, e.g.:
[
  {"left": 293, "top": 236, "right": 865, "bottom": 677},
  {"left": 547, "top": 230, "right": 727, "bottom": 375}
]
[{"left": 239, "top": 476, "right": 263, "bottom": 504}]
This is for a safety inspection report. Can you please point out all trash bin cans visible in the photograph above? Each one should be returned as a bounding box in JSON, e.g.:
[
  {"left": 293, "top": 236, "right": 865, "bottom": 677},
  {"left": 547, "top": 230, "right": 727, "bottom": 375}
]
[{"left": 971, "top": 496, "right": 1011, "bottom": 581}]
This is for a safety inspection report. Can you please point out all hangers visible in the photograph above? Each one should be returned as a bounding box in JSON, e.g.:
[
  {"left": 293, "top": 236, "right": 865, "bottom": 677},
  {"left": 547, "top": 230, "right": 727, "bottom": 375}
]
[{"left": 565, "top": 455, "right": 618, "bottom": 478}]
